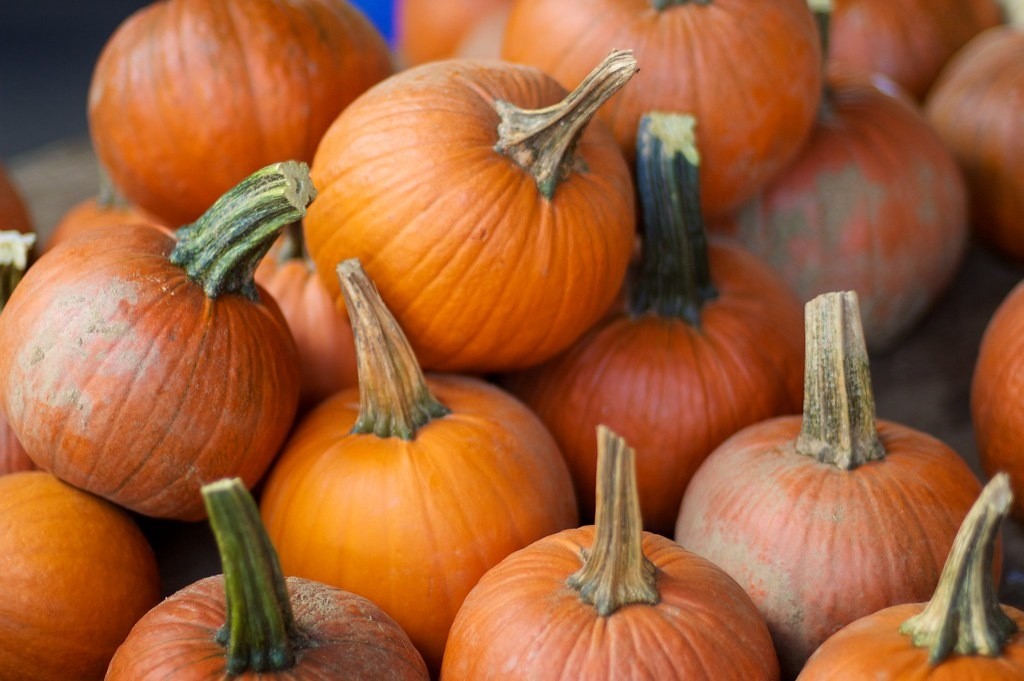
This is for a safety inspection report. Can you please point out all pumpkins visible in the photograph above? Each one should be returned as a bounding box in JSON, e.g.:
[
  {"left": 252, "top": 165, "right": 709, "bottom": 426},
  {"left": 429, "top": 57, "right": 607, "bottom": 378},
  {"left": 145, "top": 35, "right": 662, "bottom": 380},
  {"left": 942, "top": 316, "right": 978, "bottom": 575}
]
[{"left": 0, "top": 0, "right": 1024, "bottom": 681}]
